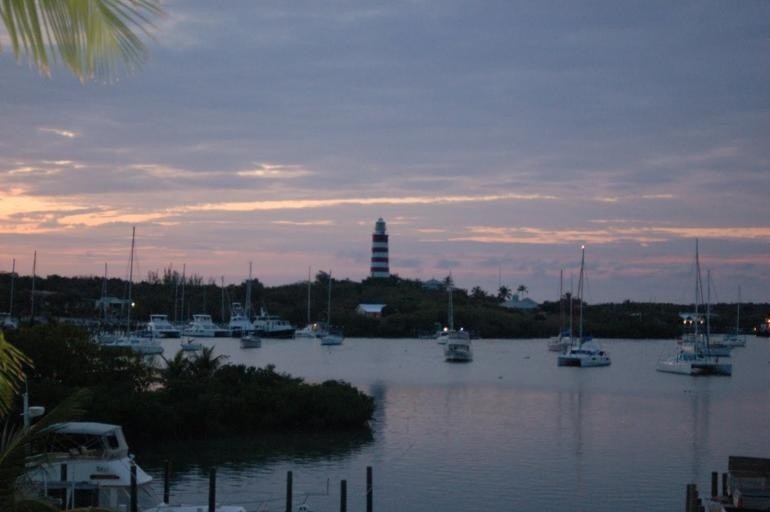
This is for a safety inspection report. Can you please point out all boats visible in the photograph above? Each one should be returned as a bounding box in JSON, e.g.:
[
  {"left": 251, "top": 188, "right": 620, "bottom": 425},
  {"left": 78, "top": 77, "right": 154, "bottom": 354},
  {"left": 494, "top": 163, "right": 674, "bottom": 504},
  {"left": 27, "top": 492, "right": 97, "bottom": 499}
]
[{"left": 10, "top": 388, "right": 331, "bottom": 511}]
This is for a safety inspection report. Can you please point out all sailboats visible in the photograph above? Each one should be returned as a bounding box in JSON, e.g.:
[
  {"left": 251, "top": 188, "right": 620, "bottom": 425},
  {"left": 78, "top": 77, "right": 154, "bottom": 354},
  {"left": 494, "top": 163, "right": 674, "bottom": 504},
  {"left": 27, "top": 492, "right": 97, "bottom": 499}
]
[
  {"left": 432, "top": 281, "right": 474, "bottom": 364},
  {"left": 655, "top": 236, "right": 770, "bottom": 378},
  {"left": 547, "top": 244, "right": 612, "bottom": 369}
]
